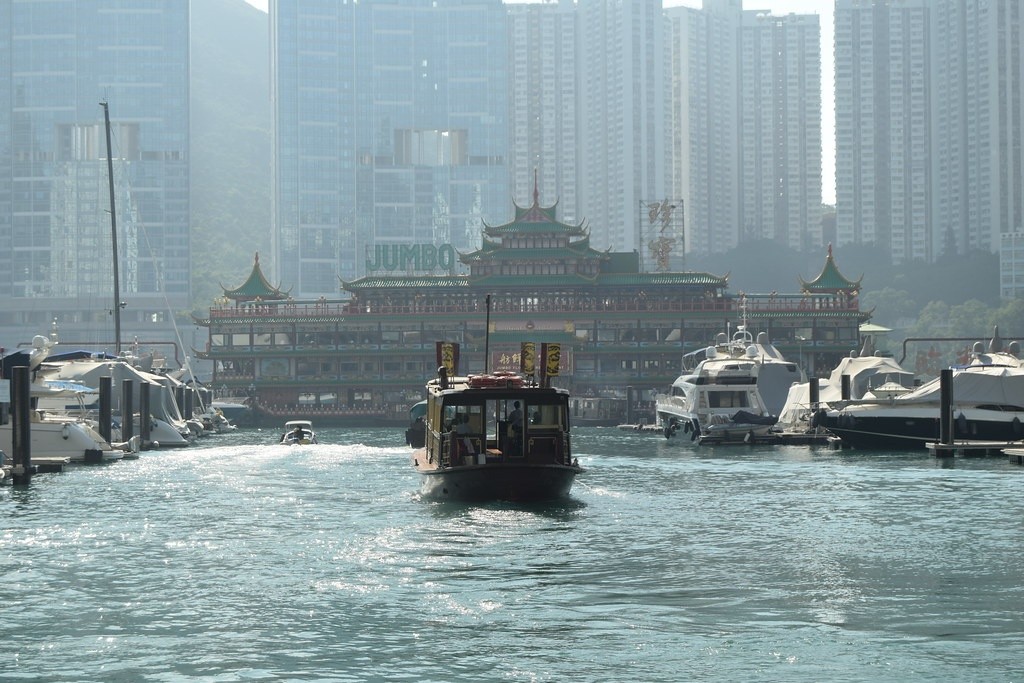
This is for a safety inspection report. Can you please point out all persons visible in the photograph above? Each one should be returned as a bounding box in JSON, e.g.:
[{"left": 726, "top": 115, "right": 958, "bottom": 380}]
[
  {"left": 531, "top": 411, "right": 541, "bottom": 424},
  {"left": 294, "top": 425, "right": 305, "bottom": 439},
  {"left": 448, "top": 416, "right": 470, "bottom": 434},
  {"left": 508, "top": 400, "right": 522, "bottom": 439}
]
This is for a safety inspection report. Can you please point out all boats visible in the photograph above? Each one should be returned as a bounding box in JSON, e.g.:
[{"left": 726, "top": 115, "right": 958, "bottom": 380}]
[
  {"left": 410, "top": 294, "right": 588, "bottom": 502},
  {"left": 1, "top": 413, "right": 124, "bottom": 464},
  {"left": 190, "top": 166, "right": 878, "bottom": 427},
  {"left": 0, "top": 348, "right": 237, "bottom": 445},
  {"left": 733, "top": 294, "right": 804, "bottom": 418},
  {"left": 279, "top": 420, "right": 317, "bottom": 445},
  {"left": 772, "top": 337, "right": 916, "bottom": 432},
  {"left": 655, "top": 320, "right": 779, "bottom": 444},
  {"left": 817, "top": 325, "right": 1024, "bottom": 451},
  {"left": 405, "top": 430, "right": 425, "bottom": 449}
]
